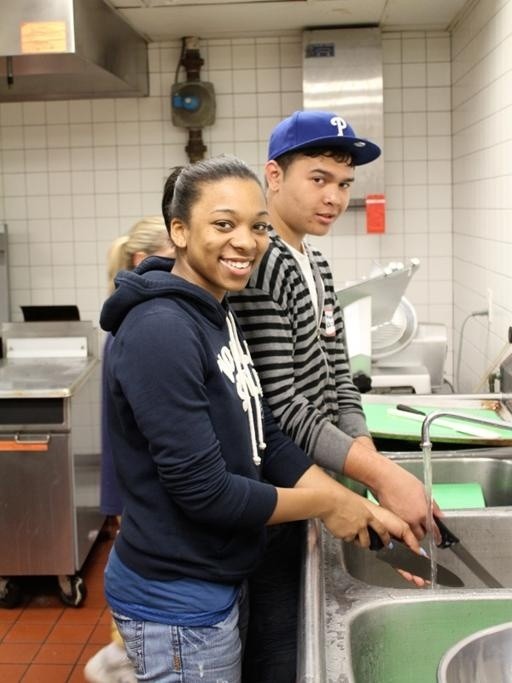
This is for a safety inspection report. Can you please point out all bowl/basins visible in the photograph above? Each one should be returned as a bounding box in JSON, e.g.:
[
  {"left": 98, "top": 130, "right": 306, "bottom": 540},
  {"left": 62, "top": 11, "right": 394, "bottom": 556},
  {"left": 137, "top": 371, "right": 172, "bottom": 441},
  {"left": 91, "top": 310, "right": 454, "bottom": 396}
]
[{"left": 435, "top": 618, "right": 512, "bottom": 683}]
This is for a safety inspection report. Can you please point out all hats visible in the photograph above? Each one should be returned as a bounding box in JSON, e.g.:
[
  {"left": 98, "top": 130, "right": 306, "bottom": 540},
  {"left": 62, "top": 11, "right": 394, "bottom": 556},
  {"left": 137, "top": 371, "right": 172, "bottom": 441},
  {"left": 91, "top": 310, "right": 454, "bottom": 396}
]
[{"left": 267, "top": 109, "right": 381, "bottom": 166}]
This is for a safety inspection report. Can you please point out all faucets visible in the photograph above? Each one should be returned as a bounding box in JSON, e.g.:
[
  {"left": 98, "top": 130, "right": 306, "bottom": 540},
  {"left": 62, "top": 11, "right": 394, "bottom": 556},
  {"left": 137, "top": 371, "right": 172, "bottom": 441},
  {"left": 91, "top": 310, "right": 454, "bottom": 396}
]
[{"left": 420, "top": 410, "right": 512, "bottom": 449}]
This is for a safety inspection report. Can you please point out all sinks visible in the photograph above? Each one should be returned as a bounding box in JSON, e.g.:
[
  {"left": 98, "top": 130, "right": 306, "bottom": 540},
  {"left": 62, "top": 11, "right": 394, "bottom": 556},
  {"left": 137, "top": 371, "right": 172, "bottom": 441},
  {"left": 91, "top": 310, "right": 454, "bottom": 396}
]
[
  {"left": 348, "top": 597, "right": 512, "bottom": 682},
  {"left": 340, "top": 512, "right": 512, "bottom": 593},
  {"left": 370, "top": 450, "right": 512, "bottom": 510}
]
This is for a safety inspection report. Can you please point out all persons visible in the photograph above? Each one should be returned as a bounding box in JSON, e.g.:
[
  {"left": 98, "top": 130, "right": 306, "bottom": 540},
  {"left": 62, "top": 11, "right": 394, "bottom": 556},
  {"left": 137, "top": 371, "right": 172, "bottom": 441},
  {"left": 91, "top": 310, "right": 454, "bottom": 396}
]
[
  {"left": 101, "top": 154, "right": 432, "bottom": 683},
  {"left": 81, "top": 211, "right": 179, "bottom": 683},
  {"left": 223, "top": 106, "right": 446, "bottom": 683}
]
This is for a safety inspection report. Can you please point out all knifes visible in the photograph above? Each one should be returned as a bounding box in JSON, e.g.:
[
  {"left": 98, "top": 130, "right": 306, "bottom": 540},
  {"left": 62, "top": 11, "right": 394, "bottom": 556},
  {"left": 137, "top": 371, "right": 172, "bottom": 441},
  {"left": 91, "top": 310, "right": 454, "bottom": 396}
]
[
  {"left": 427, "top": 512, "right": 505, "bottom": 589},
  {"left": 354, "top": 523, "right": 465, "bottom": 592}
]
